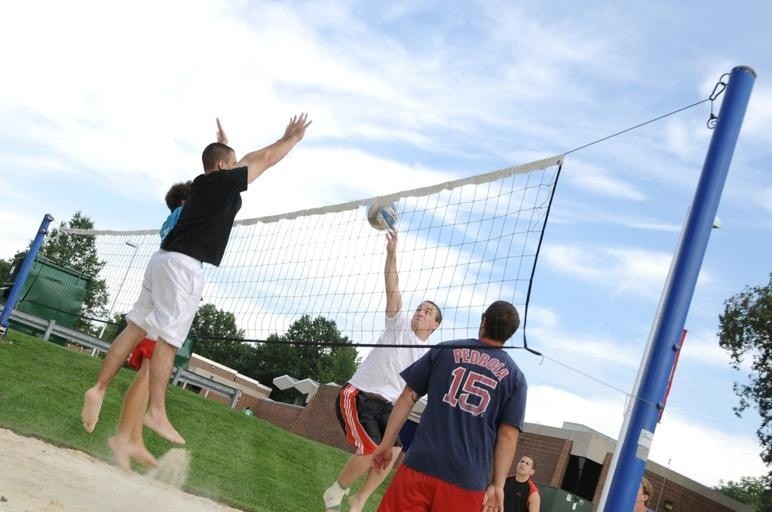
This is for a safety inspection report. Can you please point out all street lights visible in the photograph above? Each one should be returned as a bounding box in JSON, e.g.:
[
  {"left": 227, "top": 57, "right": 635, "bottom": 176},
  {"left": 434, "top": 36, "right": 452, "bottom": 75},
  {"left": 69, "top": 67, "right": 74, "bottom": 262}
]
[
  {"left": 91, "top": 241, "right": 138, "bottom": 357},
  {"left": 595, "top": 203, "right": 720, "bottom": 512}
]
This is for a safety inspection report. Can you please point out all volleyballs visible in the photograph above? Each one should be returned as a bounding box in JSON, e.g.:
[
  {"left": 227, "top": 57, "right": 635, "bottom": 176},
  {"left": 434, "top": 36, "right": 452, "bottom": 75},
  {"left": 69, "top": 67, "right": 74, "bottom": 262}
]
[{"left": 368, "top": 202, "right": 397, "bottom": 229}]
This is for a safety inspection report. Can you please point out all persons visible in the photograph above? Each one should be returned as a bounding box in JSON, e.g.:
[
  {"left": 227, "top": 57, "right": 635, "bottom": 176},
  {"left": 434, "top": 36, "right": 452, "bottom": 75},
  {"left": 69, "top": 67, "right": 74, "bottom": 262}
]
[
  {"left": 632, "top": 478, "right": 653, "bottom": 512},
  {"left": 108, "top": 116, "right": 229, "bottom": 478},
  {"left": 242, "top": 406, "right": 252, "bottom": 416},
  {"left": 323, "top": 225, "right": 443, "bottom": 512},
  {"left": 372, "top": 301, "right": 528, "bottom": 512},
  {"left": 81, "top": 113, "right": 313, "bottom": 451},
  {"left": 503, "top": 455, "right": 541, "bottom": 512}
]
